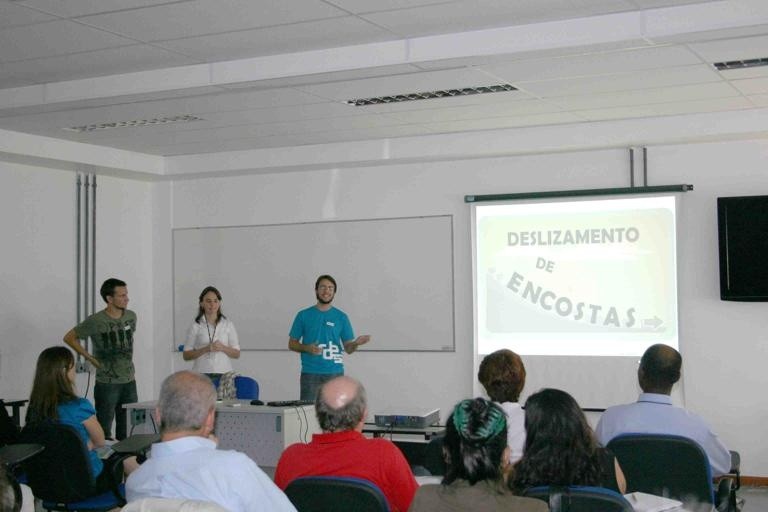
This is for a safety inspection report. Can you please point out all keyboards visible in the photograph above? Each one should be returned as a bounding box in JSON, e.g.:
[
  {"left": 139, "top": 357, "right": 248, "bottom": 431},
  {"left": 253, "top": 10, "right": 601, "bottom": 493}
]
[{"left": 267, "top": 399, "right": 315, "bottom": 406}]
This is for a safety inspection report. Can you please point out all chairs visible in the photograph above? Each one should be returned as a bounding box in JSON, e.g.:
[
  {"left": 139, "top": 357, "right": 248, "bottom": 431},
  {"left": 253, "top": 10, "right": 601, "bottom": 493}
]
[{"left": 212, "top": 375, "right": 259, "bottom": 400}]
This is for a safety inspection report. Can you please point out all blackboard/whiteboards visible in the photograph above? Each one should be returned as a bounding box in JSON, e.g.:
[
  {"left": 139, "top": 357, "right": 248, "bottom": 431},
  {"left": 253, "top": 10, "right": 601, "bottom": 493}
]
[{"left": 171, "top": 215, "right": 455, "bottom": 353}]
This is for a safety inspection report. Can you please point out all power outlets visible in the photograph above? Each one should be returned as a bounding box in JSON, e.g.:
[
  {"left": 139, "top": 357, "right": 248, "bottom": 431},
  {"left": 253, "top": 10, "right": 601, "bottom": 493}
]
[{"left": 84, "top": 362, "right": 92, "bottom": 372}]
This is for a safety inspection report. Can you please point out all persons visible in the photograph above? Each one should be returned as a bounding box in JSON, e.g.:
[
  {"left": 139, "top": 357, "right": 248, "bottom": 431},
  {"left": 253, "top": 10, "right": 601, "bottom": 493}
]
[
  {"left": 122, "top": 371, "right": 297, "bottom": 512},
  {"left": 23, "top": 343, "right": 107, "bottom": 487},
  {"left": 62, "top": 278, "right": 138, "bottom": 443},
  {"left": 289, "top": 273, "right": 372, "bottom": 403},
  {"left": 506, "top": 387, "right": 628, "bottom": 494},
  {"left": 182, "top": 285, "right": 241, "bottom": 397},
  {"left": 475, "top": 348, "right": 529, "bottom": 468},
  {"left": 278, "top": 376, "right": 420, "bottom": 512},
  {"left": 405, "top": 397, "right": 551, "bottom": 512},
  {"left": 594, "top": 344, "right": 732, "bottom": 481}
]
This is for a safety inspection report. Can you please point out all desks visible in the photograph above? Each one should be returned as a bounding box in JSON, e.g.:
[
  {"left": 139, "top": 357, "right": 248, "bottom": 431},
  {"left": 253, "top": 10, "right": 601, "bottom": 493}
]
[{"left": 121, "top": 400, "right": 324, "bottom": 467}]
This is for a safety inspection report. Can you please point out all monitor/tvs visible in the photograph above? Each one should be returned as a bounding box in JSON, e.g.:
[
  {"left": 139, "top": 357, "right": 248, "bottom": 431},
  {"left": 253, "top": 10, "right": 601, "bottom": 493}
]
[{"left": 715, "top": 193, "right": 767, "bottom": 301}]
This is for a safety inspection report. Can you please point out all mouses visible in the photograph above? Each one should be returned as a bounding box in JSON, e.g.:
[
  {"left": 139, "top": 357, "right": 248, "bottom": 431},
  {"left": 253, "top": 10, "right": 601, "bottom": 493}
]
[{"left": 251, "top": 399, "right": 263, "bottom": 405}]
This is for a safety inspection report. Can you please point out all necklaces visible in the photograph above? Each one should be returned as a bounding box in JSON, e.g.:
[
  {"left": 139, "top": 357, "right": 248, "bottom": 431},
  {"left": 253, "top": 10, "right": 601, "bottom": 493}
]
[{"left": 205, "top": 315, "right": 218, "bottom": 344}]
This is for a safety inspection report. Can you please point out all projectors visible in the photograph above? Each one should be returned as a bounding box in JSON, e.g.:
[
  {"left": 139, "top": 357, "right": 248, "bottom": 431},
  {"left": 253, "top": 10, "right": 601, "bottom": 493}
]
[{"left": 374, "top": 406, "right": 441, "bottom": 429}]
